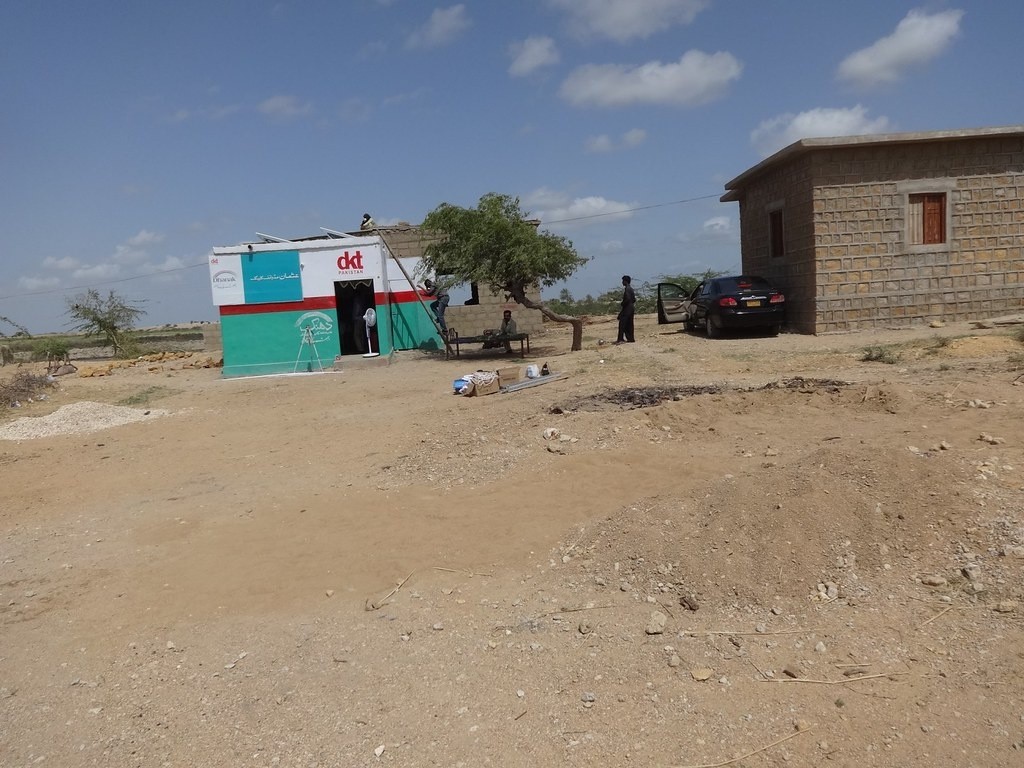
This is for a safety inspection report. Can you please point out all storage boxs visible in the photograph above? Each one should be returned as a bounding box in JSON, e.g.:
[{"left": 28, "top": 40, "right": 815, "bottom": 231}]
[
  {"left": 455, "top": 373, "right": 500, "bottom": 396},
  {"left": 478, "top": 366, "right": 521, "bottom": 389}
]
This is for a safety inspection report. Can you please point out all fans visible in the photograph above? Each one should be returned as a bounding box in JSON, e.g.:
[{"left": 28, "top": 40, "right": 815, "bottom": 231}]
[{"left": 363, "top": 307, "right": 379, "bottom": 358}]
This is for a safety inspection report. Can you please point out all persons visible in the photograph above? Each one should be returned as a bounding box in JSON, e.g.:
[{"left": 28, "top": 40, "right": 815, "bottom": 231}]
[
  {"left": 497, "top": 310, "right": 517, "bottom": 354},
  {"left": 360, "top": 214, "right": 377, "bottom": 230},
  {"left": 613, "top": 276, "right": 636, "bottom": 345},
  {"left": 417, "top": 279, "right": 450, "bottom": 334}
]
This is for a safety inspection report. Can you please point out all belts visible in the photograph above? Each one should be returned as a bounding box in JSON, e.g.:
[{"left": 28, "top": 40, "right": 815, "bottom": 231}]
[{"left": 438, "top": 295, "right": 449, "bottom": 299}]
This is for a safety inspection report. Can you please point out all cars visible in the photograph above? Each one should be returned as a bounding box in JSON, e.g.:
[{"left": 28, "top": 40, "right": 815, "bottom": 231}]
[{"left": 655, "top": 272, "right": 786, "bottom": 339}]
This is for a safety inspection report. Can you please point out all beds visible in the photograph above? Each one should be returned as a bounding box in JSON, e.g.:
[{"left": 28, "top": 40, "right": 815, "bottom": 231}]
[{"left": 445, "top": 327, "right": 529, "bottom": 360}]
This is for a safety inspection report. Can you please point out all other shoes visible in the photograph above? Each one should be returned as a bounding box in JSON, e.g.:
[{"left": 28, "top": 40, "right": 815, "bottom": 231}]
[
  {"left": 437, "top": 330, "right": 447, "bottom": 335},
  {"left": 432, "top": 319, "right": 440, "bottom": 324},
  {"left": 506, "top": 349, "right": 513, "bottom": 353}
]
